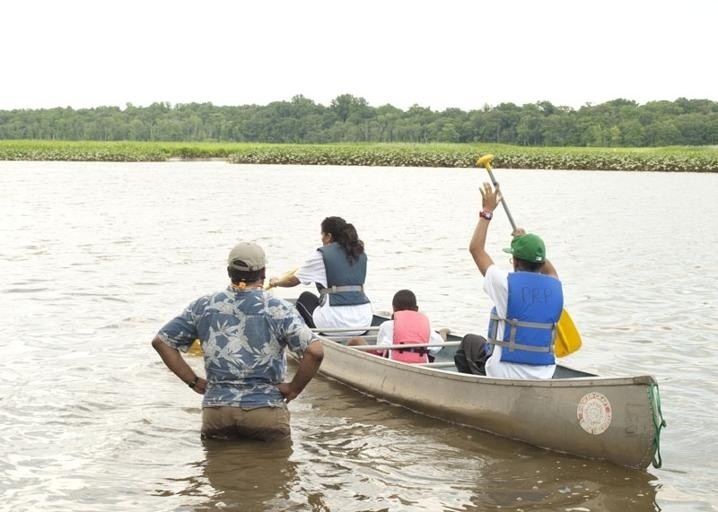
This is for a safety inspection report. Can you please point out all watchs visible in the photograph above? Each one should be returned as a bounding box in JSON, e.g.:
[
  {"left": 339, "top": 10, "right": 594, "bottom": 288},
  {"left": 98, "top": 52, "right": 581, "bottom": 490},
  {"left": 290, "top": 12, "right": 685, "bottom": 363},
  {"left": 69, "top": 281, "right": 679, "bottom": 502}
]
[{"left": 479, "top": 207, "right": 492, "bottom": 218}]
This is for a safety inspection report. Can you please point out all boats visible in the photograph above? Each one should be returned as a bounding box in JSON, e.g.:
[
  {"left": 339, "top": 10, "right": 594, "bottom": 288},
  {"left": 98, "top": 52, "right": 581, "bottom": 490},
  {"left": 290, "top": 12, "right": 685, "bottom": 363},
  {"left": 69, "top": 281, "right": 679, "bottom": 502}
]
[{"left": 278, "top": 298, "right": 659, "bottom": 469}]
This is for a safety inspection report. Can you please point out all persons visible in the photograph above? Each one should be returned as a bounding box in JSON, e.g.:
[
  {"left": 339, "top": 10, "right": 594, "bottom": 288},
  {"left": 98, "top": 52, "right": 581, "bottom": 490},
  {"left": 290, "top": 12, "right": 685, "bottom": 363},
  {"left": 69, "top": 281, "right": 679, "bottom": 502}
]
[
  {"left": 345, "top": 289, "right": 451, "bottom": 363},
  {"left": 269, "top": 215, "right": 376, "bottom": 336},
  {"left": 150, "top": 243, "right": 325, "bottom": 438},
  {"left": 455, "top": 182, "right": 564, "bottom": 380}
]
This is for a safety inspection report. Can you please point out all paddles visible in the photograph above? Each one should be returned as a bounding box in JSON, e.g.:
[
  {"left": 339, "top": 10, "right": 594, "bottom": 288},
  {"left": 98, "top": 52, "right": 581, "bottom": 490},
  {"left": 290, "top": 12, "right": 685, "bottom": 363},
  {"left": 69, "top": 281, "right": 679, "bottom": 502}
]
[
  {"left": 480, "top": 153, "right": 583, "bottom": 358},
  {"left": 190, "top": 265, "right": 299, "bottom": 359}
]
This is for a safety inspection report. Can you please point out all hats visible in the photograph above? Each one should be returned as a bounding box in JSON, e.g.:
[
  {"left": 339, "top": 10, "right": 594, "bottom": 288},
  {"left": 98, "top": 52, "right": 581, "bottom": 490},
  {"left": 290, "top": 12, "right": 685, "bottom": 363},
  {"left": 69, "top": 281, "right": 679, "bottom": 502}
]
[
  {"left": 503, "top": 234, "right": 545, "bottom": 263},
  {"left": 229, "top": 243, "right": 266, "bottom": 272}
]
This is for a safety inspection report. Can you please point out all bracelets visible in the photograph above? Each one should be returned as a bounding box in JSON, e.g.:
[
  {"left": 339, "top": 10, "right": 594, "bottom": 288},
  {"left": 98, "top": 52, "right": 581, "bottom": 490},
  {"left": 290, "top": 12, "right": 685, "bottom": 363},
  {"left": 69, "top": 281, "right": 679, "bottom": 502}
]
[{"left": 187, "top": 374, "right": 198, "bottom": 389}]
